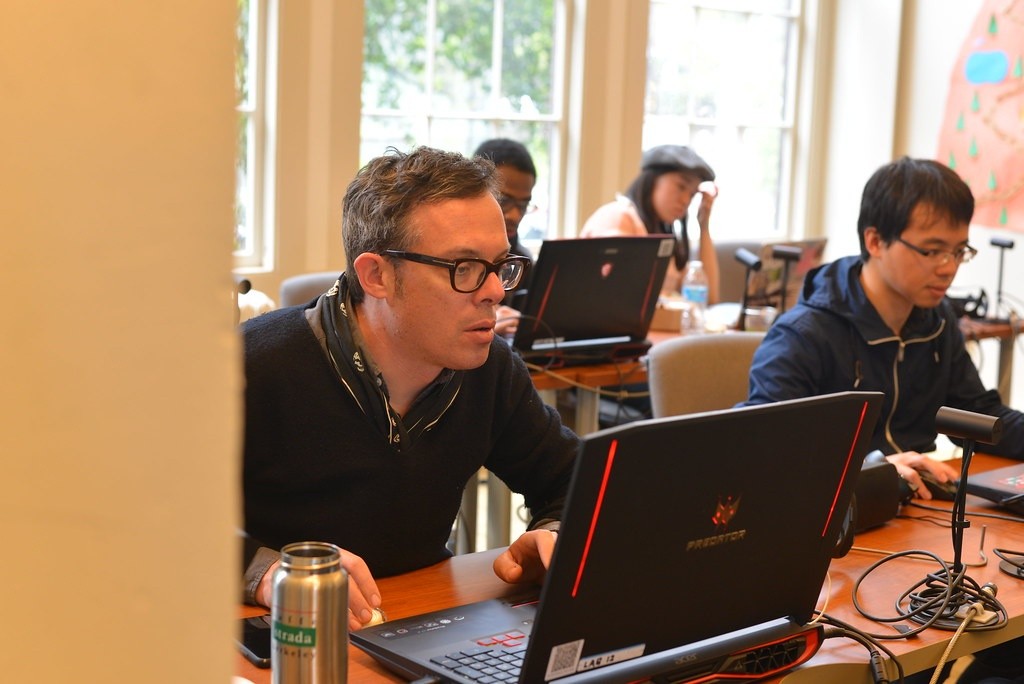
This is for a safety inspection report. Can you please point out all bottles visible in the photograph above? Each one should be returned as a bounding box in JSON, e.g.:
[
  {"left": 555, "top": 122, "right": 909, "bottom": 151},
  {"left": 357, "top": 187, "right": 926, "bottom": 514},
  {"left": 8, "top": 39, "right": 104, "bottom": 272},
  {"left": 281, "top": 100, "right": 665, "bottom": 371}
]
[{"left": 680, "top": 260, "right": 711, "bottom": 336}]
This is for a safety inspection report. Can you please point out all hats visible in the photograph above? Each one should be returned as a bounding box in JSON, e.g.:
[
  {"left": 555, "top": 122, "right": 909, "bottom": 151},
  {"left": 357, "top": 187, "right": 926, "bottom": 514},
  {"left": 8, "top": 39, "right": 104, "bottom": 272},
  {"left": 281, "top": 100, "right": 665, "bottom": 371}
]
[{"left": 642, "top": 145, "right": 715, "bottom": 181}]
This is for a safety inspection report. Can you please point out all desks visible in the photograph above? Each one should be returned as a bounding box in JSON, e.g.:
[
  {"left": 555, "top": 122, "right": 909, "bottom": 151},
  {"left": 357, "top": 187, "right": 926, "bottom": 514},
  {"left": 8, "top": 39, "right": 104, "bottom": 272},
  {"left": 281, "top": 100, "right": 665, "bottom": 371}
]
[
  {"left": 446, "top": 369, "right": 579, "bottom": 556},
  {"left": 572, "top": 289, "right": 1024, "bottom": 435},
  {"left": 233, "top": 453, "right": 1024, "bottom": 684}
]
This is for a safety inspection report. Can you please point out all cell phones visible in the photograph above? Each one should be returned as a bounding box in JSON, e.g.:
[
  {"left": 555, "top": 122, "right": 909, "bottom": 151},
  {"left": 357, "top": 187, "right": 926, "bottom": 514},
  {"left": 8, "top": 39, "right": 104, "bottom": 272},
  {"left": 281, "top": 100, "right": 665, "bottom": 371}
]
[{"left": 236, "top": 613, "right": 272, "bottom": 668}]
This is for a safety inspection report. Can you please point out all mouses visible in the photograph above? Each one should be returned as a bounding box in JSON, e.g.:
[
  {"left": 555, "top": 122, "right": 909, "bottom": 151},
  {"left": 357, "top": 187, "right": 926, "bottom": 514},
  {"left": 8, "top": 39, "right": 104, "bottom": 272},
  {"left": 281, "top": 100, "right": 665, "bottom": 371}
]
[
  {"left": 347, "top": 607, "right": 387, "bottom": 630},
  {"left": 913, "top": 468, "right": 958, "bottom": 502}
]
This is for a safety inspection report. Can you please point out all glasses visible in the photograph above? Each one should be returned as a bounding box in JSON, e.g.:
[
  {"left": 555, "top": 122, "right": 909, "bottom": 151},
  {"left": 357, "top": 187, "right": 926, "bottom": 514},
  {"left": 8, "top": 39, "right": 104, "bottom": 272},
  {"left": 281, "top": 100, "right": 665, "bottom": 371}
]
[
  {"left": 496, "top": 196, "right": 536, "bottom": 215},
  {"left": 893, "top": 236, "right": 977, "bottom": 263},
  {"left": 379, "top": 249, "right": 531, "bottom": 292}
]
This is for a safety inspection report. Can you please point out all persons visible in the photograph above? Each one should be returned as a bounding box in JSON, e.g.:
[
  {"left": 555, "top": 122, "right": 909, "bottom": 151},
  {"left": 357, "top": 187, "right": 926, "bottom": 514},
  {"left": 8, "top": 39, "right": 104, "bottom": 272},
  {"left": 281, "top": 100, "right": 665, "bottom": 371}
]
[
  {"left": 240, "top": 145, "right": 575, "bottom": 633},
  {"left": 571, "top": 145, "right": 721, "bottom": 417},
  {"left": 734, "top": 155, "right": 1024, "bottom": 497},
  {"left": 473, "top": 138, "right": 539, "bottom": 335}
]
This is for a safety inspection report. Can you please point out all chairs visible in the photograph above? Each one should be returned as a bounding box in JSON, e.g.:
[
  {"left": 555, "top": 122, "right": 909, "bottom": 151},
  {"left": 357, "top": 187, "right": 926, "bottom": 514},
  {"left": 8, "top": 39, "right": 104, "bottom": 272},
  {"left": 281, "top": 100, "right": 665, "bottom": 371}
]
[
  {"left": 280, "top": 272, "right": 343, "bottom": 310},
  {"left": 647, "top": 331, "right": 768, "bottom": 420}
]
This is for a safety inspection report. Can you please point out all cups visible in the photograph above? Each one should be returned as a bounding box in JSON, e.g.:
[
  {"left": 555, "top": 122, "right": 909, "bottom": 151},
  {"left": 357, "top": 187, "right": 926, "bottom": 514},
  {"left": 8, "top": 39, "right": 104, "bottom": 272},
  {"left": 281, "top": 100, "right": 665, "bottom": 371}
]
[{"left": 271, "top": 541, "right": 349, "bottom": 684}]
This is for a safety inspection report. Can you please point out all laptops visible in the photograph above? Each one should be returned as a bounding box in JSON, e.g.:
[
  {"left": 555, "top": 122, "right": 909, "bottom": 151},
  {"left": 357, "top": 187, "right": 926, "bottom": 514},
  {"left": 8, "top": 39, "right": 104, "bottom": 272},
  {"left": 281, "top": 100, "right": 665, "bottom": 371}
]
[
  {"left": 954, "top": 463, "right": 1024, "bottom": 517},
  {"left": 707, "top": 237, "right": 828, "bottom": 330},
  {"left": 349, "top": 390, "right": 885, "bottom": 684},
  {"left": 501, "top": 234, "right": 677, "bottom": 368}
]
